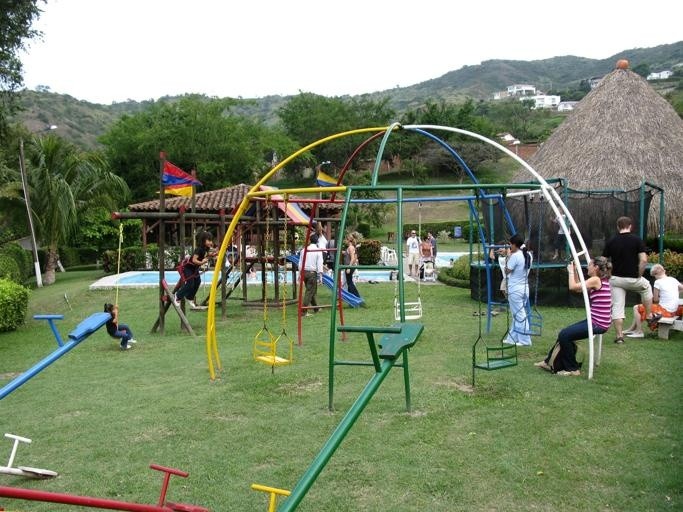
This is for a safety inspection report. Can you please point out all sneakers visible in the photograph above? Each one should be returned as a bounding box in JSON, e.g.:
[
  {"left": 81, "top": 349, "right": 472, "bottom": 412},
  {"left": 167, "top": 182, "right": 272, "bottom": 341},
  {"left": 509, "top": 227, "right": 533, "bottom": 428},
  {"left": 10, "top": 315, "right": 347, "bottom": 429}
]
[
  {"left": 622, "top": 329, "right": 645, "bottom": 338},
  {"left": 503, "top": 339, "right": 532, "bottom": 347},
  {"left": 119, "top": 338, "right": 137, "bottom": 349},
  {"left": 174, "top": 292, "right": 180, "bottom": 307},
  {"left": 187, "top": 299, "right": 196, "bottom": 308}
]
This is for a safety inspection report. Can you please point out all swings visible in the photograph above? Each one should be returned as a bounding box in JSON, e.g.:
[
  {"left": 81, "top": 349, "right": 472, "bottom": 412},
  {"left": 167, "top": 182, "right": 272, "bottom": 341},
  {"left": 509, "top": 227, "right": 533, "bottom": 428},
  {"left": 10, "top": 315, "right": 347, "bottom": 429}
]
[
  {"left": 253, "top": 191, "right": 294, "bottom": 367},
  {"left": 512, "top": 194, "right": 543, "bottom": 336},
  {"left": 176, "top": 204, "right": 277, "bottom": 283},
  {"left": 394, "top": 201, "right": 423, "bottom": 321},
  {"left": 473, "top": 186, "right": 519, "bottom": 369}
]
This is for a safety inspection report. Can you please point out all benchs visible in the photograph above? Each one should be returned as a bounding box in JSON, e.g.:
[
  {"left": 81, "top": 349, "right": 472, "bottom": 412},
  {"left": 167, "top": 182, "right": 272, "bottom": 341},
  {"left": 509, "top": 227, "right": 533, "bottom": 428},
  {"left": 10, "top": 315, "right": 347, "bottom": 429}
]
[{"left": 657, "top": 315, "right": 683, "bottom": 340}]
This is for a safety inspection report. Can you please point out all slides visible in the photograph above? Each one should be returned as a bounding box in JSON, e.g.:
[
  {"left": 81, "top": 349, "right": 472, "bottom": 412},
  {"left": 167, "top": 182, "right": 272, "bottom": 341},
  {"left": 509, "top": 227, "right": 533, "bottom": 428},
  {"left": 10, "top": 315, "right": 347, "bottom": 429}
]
[{"left": 288, "top": 255, "right": 366, "bottom": 308}]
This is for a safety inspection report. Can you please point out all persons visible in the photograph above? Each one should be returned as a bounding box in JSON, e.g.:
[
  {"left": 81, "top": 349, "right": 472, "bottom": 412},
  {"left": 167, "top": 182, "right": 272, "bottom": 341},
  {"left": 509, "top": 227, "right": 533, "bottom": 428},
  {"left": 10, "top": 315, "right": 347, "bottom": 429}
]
[
  {"left": 601, "top": 216, "right": 663, "bottom": 343},
  {"left": 406, "top": 229, "right": 437, "bottom": 279},
  {"left": 549, "top": 206, "right": 571, "bottom": 260},
  {"left": 104, "top": 302, "right": 136, "bottom": 350},
  {"left": 622, "top": 264, "right": 683, "bottom": 337},
  {"left": 174, "top": 231, "right": 214, "bottom": 307},
  {"left": 534, "top": 256, "right": 612, "bottom": 376},
  {"left": 489, "top": 234, "right": 533, "bottom": 346},
  {"left": 314, "top": 221, "right": 335, "bottom": 270},
  {"left": 450, "top": 258, "right": 453, "bottom": 265},
  {"left": 297, "top": 233, "right": 324, "bottom": 316},
  {"left": 342, "top": 231, "right": 363, "bottom": 297}
]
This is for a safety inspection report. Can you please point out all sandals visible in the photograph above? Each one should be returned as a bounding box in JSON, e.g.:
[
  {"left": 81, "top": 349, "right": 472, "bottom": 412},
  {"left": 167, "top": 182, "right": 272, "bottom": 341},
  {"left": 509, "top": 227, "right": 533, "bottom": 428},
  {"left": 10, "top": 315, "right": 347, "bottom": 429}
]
[
  {"left": 646, "top": 312, "right": 663, "bottom": 323},
  {"left": 614, "top": 337, "right": 625, "bottom": 344}
]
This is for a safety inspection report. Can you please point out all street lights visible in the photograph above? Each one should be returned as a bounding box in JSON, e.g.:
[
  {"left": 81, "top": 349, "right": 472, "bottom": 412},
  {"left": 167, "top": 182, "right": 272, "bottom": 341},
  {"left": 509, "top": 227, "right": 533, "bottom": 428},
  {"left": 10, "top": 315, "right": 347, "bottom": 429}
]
[
  {"left": 416, "top": 201, "right": 424, "bottom": 241},
  {"left": 513, "top": 139, "right": 520, "bottom": 155},
  {"left": 16, "top": 124, "right": 58, "bottom": 290},
  {"left": 317, "top": 160, "right": 331, "bottom": 200}
]
